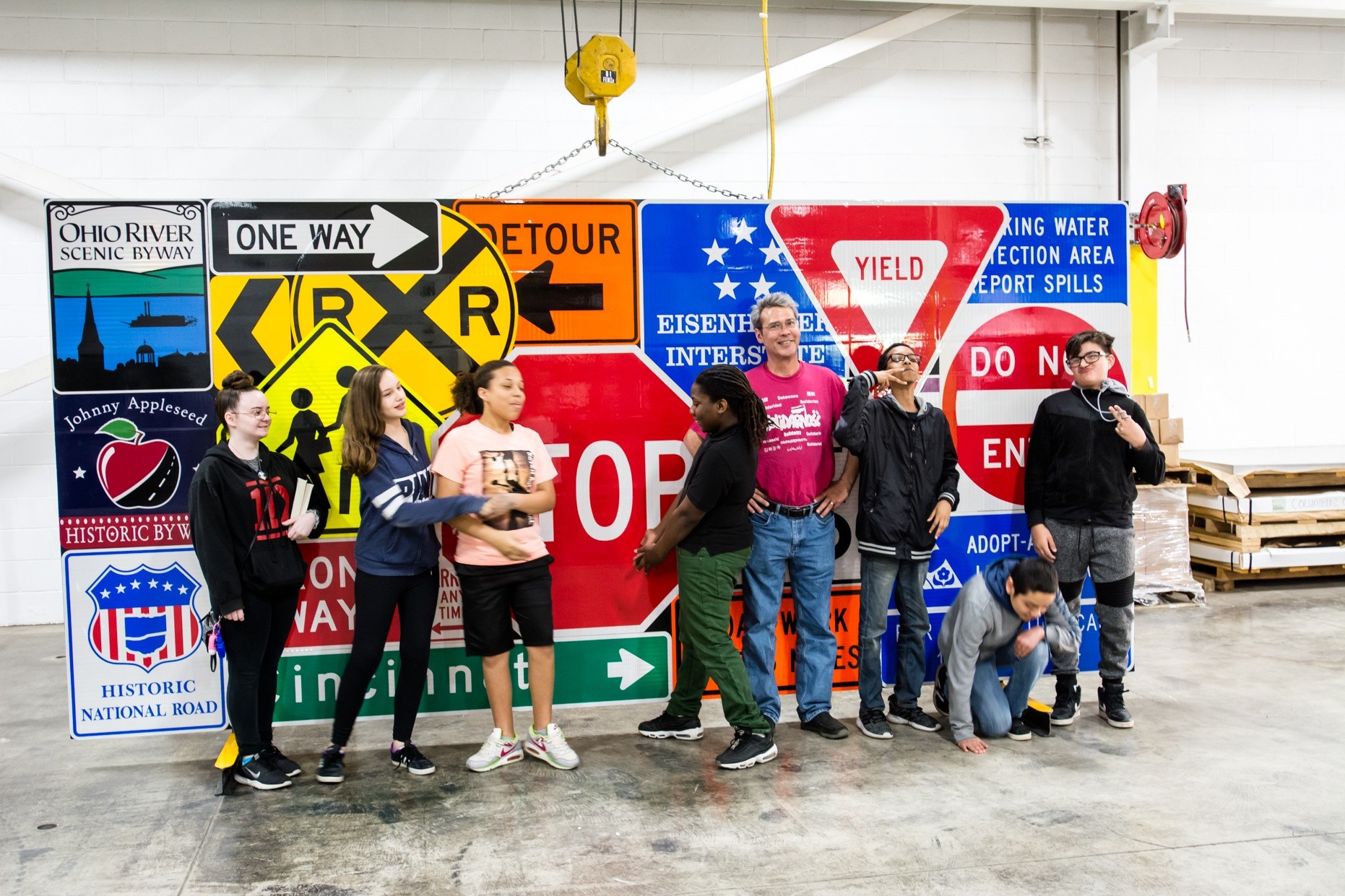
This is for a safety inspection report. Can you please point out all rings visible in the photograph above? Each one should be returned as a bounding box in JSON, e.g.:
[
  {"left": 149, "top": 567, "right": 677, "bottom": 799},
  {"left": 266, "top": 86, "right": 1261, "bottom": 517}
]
[{"left": 830, "top": 502, "right": 834, "bottom": 506}]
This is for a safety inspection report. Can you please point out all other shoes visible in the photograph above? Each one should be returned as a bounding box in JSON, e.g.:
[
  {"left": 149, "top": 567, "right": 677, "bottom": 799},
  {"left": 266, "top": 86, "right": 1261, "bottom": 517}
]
[
  {"left": 764, "top": 715, "right": 776, "bottom": 733},
  {"left": 800, "top": 712, "right": 850, "bottom": 739}
]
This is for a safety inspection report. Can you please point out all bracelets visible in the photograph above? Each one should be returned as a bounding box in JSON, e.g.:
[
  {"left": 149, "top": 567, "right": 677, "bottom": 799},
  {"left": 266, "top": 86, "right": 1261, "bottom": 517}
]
[
  {"left": 305, "top": 509, "right": 320, "bottom": 529},
  {"left": 187, "top": 371, "right": 329, "bottom": 789}
]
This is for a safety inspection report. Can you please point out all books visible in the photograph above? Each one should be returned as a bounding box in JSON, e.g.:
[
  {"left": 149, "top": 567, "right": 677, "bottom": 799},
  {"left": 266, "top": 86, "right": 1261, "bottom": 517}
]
[{"left": 288, "top": 477, "right": 314, "bottom": 532}]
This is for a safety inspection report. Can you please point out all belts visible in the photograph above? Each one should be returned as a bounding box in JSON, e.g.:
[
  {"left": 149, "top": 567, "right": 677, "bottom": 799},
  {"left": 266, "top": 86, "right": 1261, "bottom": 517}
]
[{"left": 755, "top": 497, "right": 825, "bottom": 519}]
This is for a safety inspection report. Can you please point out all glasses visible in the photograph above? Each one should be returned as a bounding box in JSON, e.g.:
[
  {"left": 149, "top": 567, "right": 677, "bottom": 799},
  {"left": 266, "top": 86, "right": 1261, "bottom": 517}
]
[
  {"left": 760, "top": 319, "right": 799, "bottom": 332},
  {"left": 230, "top": 408, "right": 278, "bottom": 420},
  {"left": 884, "top": 353, "right": 922, "bottom": 366},
  {"left": 1066, "top": 351, "right": 1109, "bottom": 368}
]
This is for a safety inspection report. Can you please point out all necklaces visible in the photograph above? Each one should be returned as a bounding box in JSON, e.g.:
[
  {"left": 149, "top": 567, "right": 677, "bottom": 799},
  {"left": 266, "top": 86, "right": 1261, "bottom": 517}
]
[{"left": 228, "top": 441, "right": 269, "bottom": 481}]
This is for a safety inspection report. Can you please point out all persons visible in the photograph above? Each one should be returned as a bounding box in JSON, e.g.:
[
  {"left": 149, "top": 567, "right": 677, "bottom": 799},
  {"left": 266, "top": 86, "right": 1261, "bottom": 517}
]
[
  {"left": 1024, "top": 330, "right": 1166, "bottom": 727},
  {"left": 834, "top": 343, "right": 960, "bottom": 737},
  {"left": 683, "top": 292, "right": 860, "bottom": 740},
  {"left": 316, "top": 364, "right": 490, "bottom": 782},
  {"left": 430, "top": 360, "right": 580, "bottom": 772},
  {"left": 633, "top": 364, "right": 779, "bottom": 768},
  {"left": 932, "top": 553, "right": 1082, "bottom": 754}
]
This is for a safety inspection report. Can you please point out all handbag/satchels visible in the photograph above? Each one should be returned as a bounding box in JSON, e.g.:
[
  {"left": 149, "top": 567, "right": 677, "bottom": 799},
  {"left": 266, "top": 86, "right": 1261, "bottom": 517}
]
[{"left": 199, "top": 610, "right": 225, "bottom": 672}]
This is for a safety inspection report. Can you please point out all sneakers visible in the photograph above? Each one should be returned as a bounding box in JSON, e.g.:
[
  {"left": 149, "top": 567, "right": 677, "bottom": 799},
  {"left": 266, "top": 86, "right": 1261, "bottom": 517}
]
[
  {"left": 857, "top": 710, "right": 894, "bottom": 739},
  {"left": 234, "top": 752, "right": 292, "bottom": 790},
  {"left": 933, "top": 663, "right": 949, "bottom": 717},
  {"left": 717, "top": 725, "right": 778, "bottom": 769},
  {"left": 314, "top": 745, "right": 346, "bottom": 783},
  {"left": 1007, "top": 717, "right": 1032, "bottom": 741},
  {"left": 1050, "top": 681, "right": 1082, "bottom": 725},
  {"left": 1098, "top": 683, "right": 1135, "bottom": 728},
  {"left": 390, "top": 740, "right": 436, "bottom": 775},
  {"left": 887, "top": 707, "right": 943, "bottom": 730},
  {"left": 638, "top": 711, "right": 704, "bottom": 740},
  {"left": 262, "top": 746, "right": 302, "bottom": 777},
  {"left": 524, "top": 723, "right": 580, "bottom": 771},
  {"left": 465, "top": 727, "right": 525, "bottom": 772}
]
